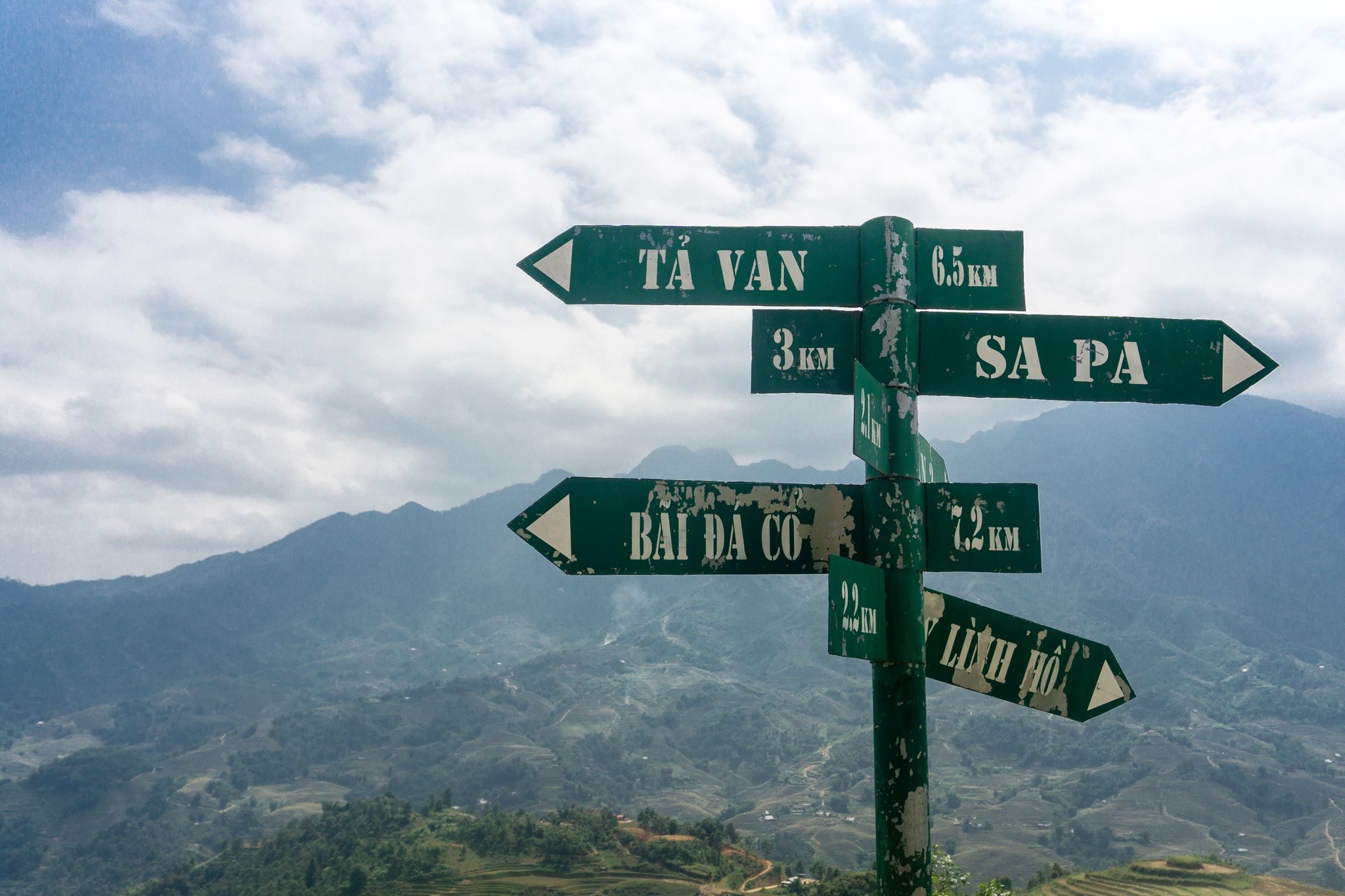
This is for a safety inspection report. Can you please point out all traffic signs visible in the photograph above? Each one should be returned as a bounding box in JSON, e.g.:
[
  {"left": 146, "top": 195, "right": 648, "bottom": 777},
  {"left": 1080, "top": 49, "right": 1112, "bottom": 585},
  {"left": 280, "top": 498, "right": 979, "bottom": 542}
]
[
  {"left": 747, "top": 302, "right": 1279, "bottom": 407},
  {"left": 512, "top": 218, "right": 1028, "bottom": 313},
  {"left": 507, "top": 475, "right": 1044, "bottom": 576},
  {"left": 825, "top": 552, "right": 1135, "bottom": 725}
]
